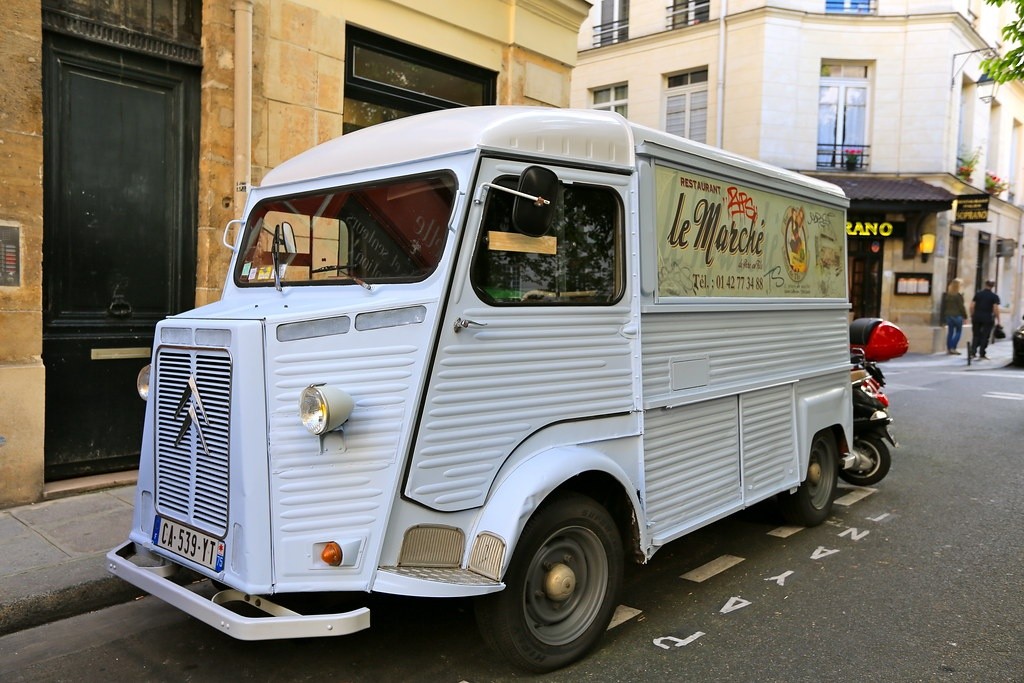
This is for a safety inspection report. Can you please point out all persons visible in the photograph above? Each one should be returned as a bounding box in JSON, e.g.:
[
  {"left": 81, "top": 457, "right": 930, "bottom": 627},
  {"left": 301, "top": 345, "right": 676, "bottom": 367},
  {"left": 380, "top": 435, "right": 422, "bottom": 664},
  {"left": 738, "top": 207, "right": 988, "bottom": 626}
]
[
  {"left": 969, "top": 279, "right": 1000, "bottom": 360},
  {"left": 939, "top": 277, "right": 968, "bottom": 355}
]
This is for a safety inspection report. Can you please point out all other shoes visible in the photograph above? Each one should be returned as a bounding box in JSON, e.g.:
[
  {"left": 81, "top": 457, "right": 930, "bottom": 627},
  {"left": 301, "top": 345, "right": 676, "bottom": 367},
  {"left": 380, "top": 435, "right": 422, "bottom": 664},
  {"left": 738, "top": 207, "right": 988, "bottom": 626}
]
[
  {"left": 947, "top": 349, "right": 961, "bottom": 354},
  {"left": 969, "top": 354, "right": 977, "bottom": 358},
  {"left": 979, "top": 356, "right": 989, "bottom": 360}
]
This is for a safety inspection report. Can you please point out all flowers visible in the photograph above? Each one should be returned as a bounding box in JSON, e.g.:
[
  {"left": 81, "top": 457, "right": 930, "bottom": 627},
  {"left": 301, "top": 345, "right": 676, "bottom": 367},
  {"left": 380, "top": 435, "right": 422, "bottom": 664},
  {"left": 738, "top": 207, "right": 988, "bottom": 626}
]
[
  {"left": 957, "top": 157, "right": 977, "bottom": 176},
  {"left": 985, "top": 172, "right": 1007, "bottom": 192},
  {"left": 844, "top": 148, "right": 863, "bottom": 164}
]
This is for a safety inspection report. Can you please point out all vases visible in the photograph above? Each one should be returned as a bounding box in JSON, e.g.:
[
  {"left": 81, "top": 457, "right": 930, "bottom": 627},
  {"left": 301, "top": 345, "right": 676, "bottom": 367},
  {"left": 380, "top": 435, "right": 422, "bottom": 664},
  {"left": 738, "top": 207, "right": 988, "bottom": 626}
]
[
  {"left": 847, "top": 161, "right": 858, "bottom": 171},
  {"left": 959, "top": 174, "right": 970, "bottom": 182},
  {"left": 987, "top": 188, "right": 995, "bottom": 194}
]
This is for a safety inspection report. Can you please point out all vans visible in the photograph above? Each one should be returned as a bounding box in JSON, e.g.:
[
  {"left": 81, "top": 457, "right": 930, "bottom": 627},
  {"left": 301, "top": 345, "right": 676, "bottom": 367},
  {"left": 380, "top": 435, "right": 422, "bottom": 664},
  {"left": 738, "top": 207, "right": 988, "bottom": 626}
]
[{"left": 106, "top": 105, "right": 856, "bottom": 672}]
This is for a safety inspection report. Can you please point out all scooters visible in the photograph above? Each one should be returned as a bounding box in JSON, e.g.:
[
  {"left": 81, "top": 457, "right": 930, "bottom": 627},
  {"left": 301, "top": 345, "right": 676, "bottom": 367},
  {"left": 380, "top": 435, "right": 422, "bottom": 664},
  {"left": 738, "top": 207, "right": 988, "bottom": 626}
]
[{"left": 837, "top": 318, "right": 909, "bottom": 487}]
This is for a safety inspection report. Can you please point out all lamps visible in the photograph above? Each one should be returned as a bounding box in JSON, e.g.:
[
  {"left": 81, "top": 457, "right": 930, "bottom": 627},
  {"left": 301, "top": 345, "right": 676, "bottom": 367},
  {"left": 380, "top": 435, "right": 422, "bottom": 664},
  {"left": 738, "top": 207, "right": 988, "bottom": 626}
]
[{"left": 919, "top": 234, "right": 934, "bottom": 262}]
